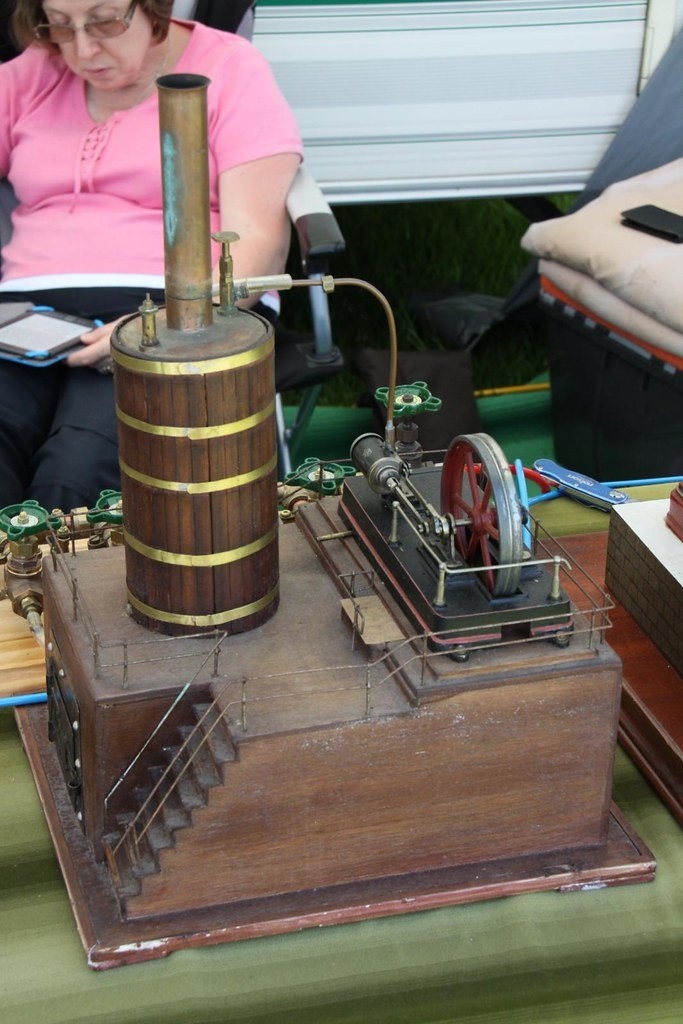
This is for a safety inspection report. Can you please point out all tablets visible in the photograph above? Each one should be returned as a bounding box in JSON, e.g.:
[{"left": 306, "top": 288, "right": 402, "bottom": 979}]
[{"left": 0, "top": 308, "right": 101, "bottom": 361}]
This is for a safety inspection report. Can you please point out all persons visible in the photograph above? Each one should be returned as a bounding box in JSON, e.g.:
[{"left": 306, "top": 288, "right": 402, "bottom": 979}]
[{"left": 0, "top": 0, "right": 304, "bottom": 516}]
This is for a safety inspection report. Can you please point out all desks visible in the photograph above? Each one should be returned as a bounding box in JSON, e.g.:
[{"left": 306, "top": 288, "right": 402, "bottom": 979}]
[{"left": 0, "top": 482, "right": 683, "bottom": 1024}]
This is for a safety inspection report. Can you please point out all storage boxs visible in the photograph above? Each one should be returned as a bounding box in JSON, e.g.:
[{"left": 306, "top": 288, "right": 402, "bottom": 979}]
[{"left": 541, "top": 286, "right": 683, "bottom": 481}]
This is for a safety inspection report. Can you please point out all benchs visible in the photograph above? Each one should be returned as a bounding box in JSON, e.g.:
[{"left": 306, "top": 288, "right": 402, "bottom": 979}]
[{"left": 280, "top": 190, "right": 588, "bottom": 470}]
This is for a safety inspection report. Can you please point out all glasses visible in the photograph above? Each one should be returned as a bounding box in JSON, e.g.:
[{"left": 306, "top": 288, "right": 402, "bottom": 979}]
[{"left": 34, "top": 0, "right": 137, "bottom": 43}]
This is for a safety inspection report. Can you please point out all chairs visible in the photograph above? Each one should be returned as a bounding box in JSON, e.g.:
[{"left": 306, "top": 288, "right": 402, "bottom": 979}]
[{"left": 276, "top": 164, "right": 346, "bottom": 480}]
